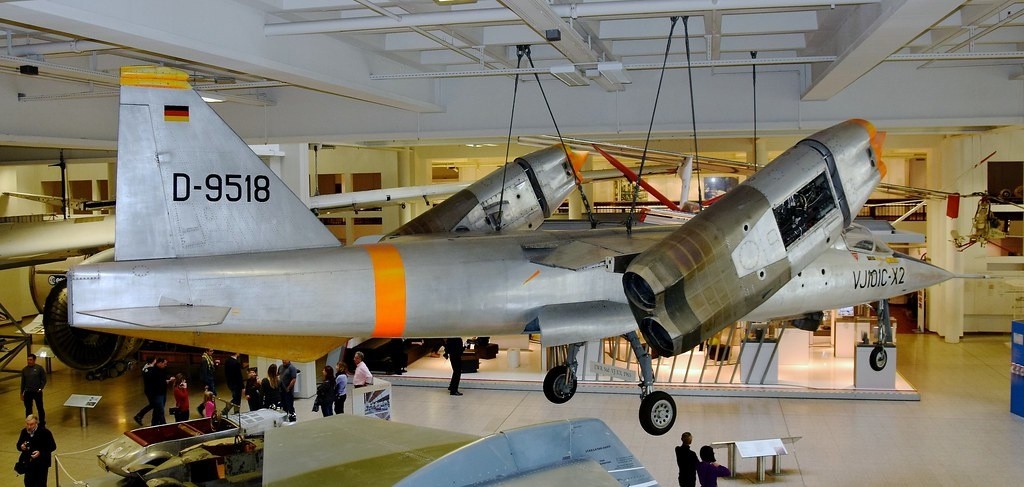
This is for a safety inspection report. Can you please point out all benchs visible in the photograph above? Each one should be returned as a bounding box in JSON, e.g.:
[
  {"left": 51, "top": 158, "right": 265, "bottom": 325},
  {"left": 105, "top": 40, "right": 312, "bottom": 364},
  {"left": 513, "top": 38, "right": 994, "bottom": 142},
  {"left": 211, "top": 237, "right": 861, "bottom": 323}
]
[{"left": 178, "top": 421, "right": 205, "bottom": 437}]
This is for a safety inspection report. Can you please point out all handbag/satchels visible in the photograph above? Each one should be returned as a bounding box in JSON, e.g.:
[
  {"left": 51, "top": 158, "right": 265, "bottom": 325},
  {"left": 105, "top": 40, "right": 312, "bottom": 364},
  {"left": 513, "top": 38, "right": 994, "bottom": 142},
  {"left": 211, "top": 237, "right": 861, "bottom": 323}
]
[
  {"left": 13, "top": 451, "right": 26, "bottom": 475},
  {"left": 169, "top": 407, "right": 181, "bottom": 415}
]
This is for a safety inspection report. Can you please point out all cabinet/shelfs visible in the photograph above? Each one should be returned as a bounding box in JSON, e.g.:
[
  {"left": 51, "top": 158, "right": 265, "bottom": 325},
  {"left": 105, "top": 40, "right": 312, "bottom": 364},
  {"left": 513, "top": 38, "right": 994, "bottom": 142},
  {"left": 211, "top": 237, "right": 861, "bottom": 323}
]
[
  {"left": 833, "top": 317, "right": 897, "bottom": 358},
  {"left": 739, "top": 339, "right": 779, "bottom": 385},
  {"left": 248, "top": 356, "right": 317, "bottom": 399},
  {"left": 614, "top": 178, "right": 648, "bottom": 202},
  {"left": 854, "top": 342, "right": 897, "bottom": 390},
  {"left": 774, "top": 328, "right": 813, "bottom": 364}
]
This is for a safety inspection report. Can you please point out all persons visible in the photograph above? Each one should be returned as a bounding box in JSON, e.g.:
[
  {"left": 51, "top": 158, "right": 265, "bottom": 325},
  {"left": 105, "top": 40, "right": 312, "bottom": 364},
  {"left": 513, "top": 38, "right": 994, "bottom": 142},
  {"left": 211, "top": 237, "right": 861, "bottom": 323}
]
[
  {"left": 260, "top": 364, "right": 290, "bottom": 410},
  {"left": 697, "top": 446, "right": 731, "bottom": 487},
  {"left": 20, "top": 353, "right": 47, "bottom": 428},
  {"left": 133, "top": 347, "right": 217, "bottom": 427},
  {"left": 221, "top": 353, "right": 245, "bottom": 418},
  {"left": 352, "top": 352, "right": 373, "bottom": 388},
  {"left": 275, "top": 359, "right": 297, "bottom": 421},
  {"left": 675, "top": 432, "right": 700, "bottom": 487},
  {"left": 245, "top": 367, "right": 263, "bottom": 411},
  {"left": 316, "top": 366, "right": 336, "bottom": 417},
  {"left": 16, "top": 415, "right": 56, "bottom": 487},
  {"left": 444, "top": 337, "right": 464, "bottom": 395},
  {"left": 334, "top": 362, "right": 347, "bottom": 414}
]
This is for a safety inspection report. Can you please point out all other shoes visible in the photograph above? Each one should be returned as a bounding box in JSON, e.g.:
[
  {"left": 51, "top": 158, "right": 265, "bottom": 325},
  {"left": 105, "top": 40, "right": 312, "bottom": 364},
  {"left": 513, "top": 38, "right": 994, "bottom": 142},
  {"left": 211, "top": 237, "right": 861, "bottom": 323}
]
[
  {"left": 133, "top": 415, "right": 142, "bottom": 426},
  {"left": 198, "top": 408, "right": 204, "bottom": 417},
  {"left": 450, "top": 390, "right": 463, "bottom": 395}
]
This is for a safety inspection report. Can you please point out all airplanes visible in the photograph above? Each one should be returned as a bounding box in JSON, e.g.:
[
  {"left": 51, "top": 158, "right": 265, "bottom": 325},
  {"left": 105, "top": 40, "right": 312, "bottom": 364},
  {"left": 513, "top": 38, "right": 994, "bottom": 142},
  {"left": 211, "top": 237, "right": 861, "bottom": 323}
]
[{"left": 0, "top": 62, "right": 993, "bottom": 436}]
